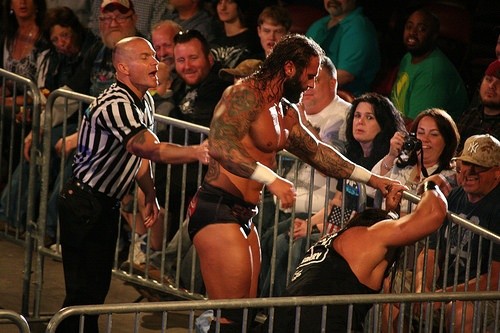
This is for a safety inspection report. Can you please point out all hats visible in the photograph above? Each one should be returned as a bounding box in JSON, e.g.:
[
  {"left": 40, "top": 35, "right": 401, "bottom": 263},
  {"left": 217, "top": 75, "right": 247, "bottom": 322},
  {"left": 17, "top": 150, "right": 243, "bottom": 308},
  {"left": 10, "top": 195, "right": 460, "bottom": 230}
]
[
  {"left": 486, "top": 60, "right": 500, "bottom": 79},
  {"left": 101, "top": 0, "right": 135, "bottom": 13},
  {"left": 218, "top": 59, "right": 262, "bottom": 80},
  {"left": 451, "top": 134, "right": 500, "bottom": 167}
]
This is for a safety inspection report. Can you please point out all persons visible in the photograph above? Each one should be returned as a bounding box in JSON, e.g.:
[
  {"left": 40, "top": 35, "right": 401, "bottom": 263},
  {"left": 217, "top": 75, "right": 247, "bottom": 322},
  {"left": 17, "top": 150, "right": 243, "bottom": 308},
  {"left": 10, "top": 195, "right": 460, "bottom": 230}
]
[
  {"left": 250, "top": 173, "right": 453, "bottom": 333},
  {"left": 186, "top": 33, "right": 400, "bottom": 333},
  {"left": 56, "top": 37, "right": 211, "bottom": 333},
  {"left": 0, "top": 0, "right": 500, "bottom": 333}
]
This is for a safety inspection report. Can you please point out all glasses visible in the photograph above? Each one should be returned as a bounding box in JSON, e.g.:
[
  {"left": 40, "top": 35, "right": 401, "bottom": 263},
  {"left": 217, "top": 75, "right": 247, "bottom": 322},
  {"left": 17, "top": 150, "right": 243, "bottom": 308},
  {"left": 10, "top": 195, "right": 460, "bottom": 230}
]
[{"left": 101, "top": 15, "right": 132, "bottom": 24}]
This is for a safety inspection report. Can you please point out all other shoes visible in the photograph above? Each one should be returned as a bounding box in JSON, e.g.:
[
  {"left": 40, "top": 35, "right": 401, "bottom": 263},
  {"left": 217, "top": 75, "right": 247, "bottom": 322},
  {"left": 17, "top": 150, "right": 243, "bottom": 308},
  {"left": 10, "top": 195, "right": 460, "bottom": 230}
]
[{"left": 23, "top": 228, "right": 204, "bottom": 316}]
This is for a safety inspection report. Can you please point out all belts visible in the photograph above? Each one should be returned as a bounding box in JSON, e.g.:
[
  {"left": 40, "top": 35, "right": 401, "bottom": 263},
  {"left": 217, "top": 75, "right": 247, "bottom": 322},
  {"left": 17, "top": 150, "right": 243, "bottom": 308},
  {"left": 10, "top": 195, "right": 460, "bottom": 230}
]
[{"left": 71, "top": 177, "right": 118, "bottom": 210}]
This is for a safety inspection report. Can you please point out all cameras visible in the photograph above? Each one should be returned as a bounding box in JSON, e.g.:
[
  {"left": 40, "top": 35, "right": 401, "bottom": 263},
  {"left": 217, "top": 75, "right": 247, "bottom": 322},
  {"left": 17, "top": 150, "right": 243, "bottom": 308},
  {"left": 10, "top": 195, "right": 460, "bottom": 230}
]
[{"left": 395, "top": 135, "right": 422, "bottom": 169}]
